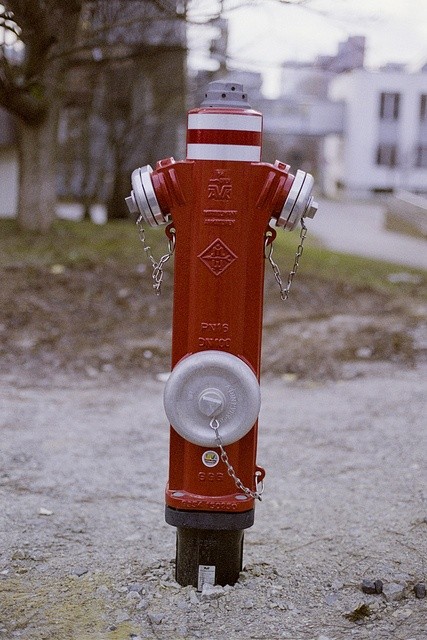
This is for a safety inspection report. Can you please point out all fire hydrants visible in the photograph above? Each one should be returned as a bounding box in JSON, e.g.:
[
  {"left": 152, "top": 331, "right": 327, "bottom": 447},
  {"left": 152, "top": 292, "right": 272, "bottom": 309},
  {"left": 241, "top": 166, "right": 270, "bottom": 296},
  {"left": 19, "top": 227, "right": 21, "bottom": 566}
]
[{"left": 125, "top": 80, "right": 319, "bottom": 590}]
